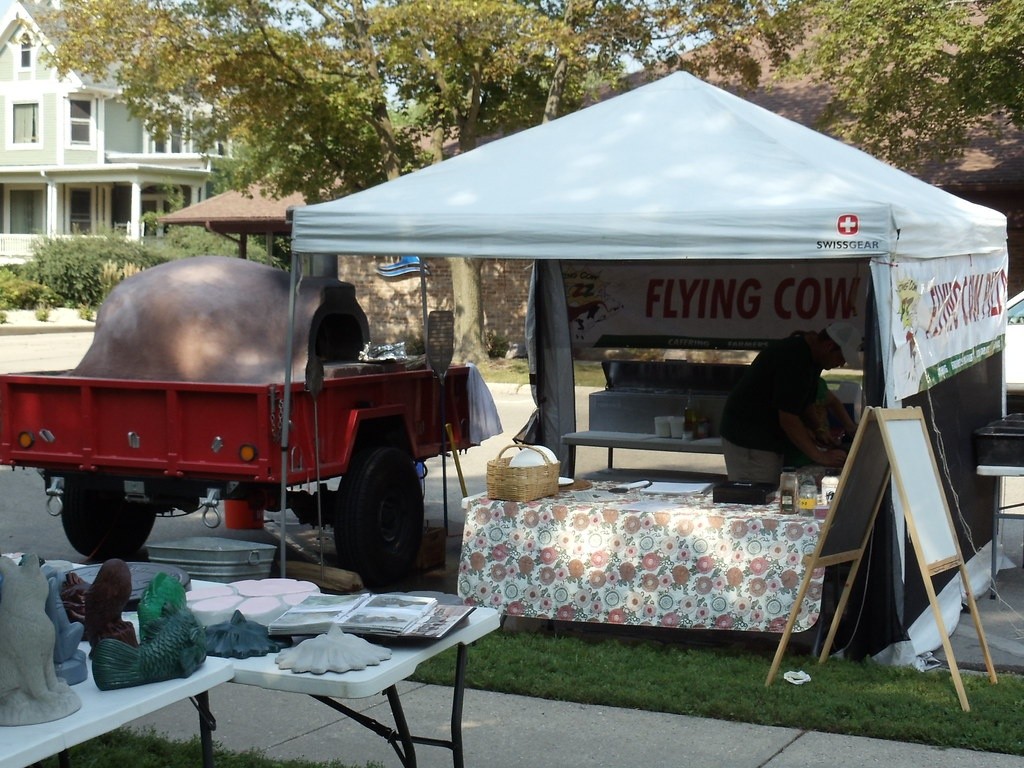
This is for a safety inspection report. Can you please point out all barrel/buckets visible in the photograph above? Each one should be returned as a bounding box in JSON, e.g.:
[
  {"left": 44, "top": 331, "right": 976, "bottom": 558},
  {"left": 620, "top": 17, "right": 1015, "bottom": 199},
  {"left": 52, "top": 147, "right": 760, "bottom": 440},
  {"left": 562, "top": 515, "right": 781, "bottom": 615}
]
[{"left": 225, "top": 499, "right": 264, "bottom": 529}]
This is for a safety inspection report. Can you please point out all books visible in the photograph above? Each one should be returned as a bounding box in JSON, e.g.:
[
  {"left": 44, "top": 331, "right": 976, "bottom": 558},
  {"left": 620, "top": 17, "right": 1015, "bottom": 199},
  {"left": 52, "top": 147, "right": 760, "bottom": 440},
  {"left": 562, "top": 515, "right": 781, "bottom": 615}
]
[
  {"left": 641, "top": 481, "right": 714, "bottom": 497},
  {"left": 267, "top": 589, "right": 478, "bottom": 638}
]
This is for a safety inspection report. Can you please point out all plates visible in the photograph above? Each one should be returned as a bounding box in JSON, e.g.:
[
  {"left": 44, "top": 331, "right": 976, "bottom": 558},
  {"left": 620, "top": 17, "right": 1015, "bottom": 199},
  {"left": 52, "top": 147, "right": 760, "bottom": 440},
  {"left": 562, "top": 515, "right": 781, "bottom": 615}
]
[
  {"left": 559, "top": 477, "right": 574, "bottom": 486},
  {"left": 509, "top": 445, "right": 557, "bottom": 467}
]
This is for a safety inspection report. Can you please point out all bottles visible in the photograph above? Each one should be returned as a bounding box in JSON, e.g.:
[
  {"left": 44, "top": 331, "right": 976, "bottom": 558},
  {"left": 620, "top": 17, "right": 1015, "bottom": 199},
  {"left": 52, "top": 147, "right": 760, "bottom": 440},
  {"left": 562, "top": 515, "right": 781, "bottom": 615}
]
[
  {"left": 684, "top": 400, "right": 696, "bottom": 440},
  {"left": 821, "top": 470, "right": 839, "bottom": 506},
  {"left": 780, "top": 467, "right": 799, "bottom": 513}
]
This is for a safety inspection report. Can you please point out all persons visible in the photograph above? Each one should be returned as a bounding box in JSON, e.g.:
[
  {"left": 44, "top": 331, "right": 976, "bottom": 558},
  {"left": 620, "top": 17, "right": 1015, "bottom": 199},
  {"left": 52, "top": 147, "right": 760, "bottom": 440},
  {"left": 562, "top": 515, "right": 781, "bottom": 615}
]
[{"left": 716, "top": 323, "right": 860, "bottom": 483}]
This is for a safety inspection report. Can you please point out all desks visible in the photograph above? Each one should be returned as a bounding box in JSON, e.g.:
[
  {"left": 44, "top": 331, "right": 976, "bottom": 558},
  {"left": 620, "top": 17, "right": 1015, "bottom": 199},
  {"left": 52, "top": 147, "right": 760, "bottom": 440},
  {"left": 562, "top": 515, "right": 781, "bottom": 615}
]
[
  {"left": 0, "top": 560, "right": 501, "bottom": 768},
  {"left": 456, "top": 476, "right": 826, "bottom": 660},
  {"left": 560, "top": 430, "right": 730, "bottom": 494}
]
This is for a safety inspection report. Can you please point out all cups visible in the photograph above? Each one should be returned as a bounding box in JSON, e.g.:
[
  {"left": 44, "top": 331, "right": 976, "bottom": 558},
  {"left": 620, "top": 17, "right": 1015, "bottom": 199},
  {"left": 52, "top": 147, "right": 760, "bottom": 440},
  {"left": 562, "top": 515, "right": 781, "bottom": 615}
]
[
  {"left": 799, "top": 485, "right": 817, "bottom": 517},
  {"left": 655, "top": 416, "right": 670, "bottom": 437},
  {"left": 670, "top": 416, "right": 684, "bottom": 439}
]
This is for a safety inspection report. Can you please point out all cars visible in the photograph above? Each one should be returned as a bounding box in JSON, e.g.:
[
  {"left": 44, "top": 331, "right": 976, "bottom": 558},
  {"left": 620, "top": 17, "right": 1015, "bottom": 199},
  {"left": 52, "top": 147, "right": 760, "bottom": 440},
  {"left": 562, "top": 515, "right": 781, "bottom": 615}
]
[{"left": 1006, "top": 292, "right": 1024, "bottom": 394}]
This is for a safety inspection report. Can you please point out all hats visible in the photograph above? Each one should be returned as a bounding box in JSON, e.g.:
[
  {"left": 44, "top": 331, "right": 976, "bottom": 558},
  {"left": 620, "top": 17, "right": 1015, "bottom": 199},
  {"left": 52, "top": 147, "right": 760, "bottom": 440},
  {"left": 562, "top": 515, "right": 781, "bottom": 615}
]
[{"left": 826, "top": 322, "right": 863, "bottom": 369}]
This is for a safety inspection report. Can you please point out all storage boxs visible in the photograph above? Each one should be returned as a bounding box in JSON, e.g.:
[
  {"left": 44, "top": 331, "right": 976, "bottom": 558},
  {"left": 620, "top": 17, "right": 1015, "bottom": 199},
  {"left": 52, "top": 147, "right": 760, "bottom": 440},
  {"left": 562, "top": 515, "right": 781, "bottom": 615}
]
[
  {"left": 147, "top": 536, "right": 278, "bottom": 591},
  {"left": 418, "top": 526, "right": 446, "bottom": 575}
]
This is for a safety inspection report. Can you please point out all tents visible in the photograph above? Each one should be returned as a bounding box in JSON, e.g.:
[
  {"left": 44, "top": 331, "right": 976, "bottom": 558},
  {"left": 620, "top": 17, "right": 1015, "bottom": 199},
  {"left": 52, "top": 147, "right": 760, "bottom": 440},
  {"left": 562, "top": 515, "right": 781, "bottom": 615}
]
[{"left": 278, "top": 71, "right": 1010, "bottom": 632}]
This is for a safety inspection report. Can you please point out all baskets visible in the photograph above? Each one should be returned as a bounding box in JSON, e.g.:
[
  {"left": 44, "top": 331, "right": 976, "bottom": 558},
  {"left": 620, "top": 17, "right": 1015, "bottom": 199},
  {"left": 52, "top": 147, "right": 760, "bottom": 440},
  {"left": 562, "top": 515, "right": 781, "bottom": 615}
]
[{"left": 486, "top": 444, "right": 561, "bottom": 503}]
